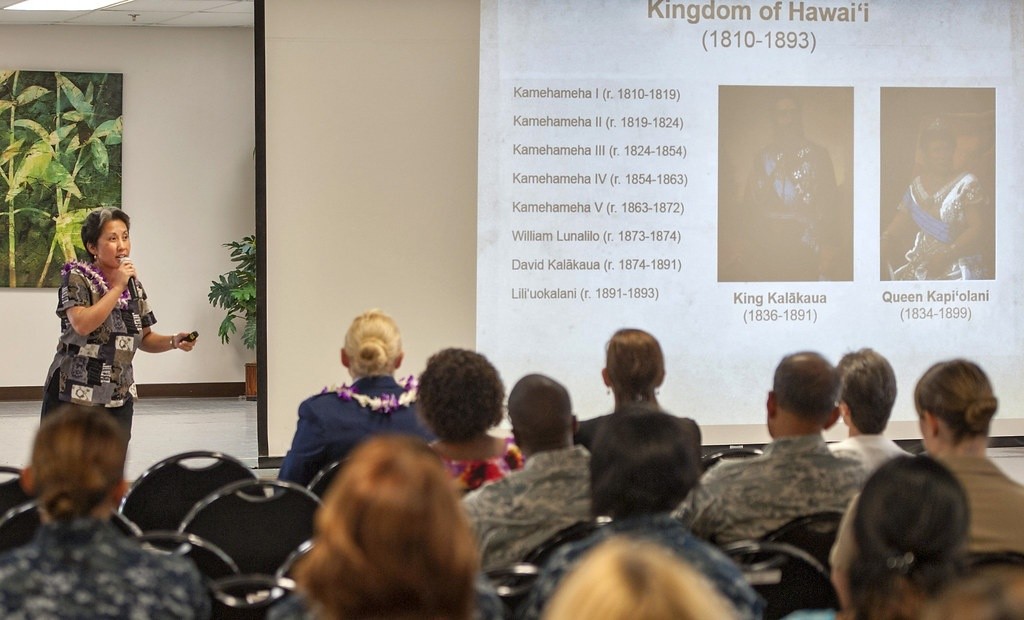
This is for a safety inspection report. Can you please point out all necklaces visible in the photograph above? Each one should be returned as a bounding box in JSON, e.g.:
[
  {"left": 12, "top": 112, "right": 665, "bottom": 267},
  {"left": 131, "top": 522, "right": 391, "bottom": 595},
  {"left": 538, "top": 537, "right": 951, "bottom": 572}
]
[
  {"left": 318, "top": 373, "right": 419, "bottom": 412},
  {"left": 60, "top": 260, "right": 132, "bottom": 310},
  {"left": 447, "top": 439, "right": 525, "bottom": 496}
]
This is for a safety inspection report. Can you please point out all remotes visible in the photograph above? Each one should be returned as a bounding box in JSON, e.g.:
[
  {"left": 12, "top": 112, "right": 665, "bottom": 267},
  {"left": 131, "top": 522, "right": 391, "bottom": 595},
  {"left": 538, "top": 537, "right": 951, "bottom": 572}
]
[{"left": 179, "top": 331, "right": 199, "bottom": 343}]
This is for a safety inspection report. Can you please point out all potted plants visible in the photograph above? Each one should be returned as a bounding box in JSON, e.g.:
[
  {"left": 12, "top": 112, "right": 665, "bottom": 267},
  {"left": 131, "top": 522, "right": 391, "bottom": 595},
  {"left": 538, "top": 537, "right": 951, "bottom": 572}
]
[{"left": 208, "top": 233, "right": 258, "bottom": 401}]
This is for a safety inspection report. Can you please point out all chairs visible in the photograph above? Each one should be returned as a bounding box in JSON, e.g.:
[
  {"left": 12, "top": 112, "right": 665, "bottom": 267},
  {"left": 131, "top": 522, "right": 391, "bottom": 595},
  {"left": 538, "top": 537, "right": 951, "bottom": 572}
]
[{"left": 0, "top": 447, "right": 1024, "bottom": 620}]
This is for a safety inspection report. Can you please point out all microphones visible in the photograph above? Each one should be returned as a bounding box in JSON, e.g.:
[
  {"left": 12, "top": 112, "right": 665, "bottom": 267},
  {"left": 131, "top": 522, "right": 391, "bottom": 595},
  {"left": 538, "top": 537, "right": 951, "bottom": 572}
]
[{"left": 120, "top": 257, "right": 139, "bottom": 301}]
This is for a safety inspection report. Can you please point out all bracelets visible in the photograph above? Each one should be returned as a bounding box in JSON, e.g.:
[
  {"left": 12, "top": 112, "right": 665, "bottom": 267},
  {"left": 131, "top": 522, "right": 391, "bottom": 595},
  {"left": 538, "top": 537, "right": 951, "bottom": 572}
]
[{"left": 170, "top": 335, "right": 177, "bottom": 349}]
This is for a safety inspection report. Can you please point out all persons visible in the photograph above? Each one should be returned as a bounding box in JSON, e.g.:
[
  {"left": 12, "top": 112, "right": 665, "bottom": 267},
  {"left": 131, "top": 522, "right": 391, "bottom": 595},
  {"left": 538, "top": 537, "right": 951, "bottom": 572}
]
[
  {"left": 41, "top": 207, "right": 197, "bottom": 462},
  {"left": 457, "top": 373, "right": 592, "bottom": 569},
  {"left": 539, "top": 533, "right": 741, "bottom": 618},
  {"left": 914, "top": 360, "right": 1023, "bottom": 552},
  {"left": 824, "top": 348, "right": 919, "bottom": 469},
  {"left": 666, "top": 353, "right": 869, "bottom": 546},
  {"left": 415, "top": 348, "right": 529, "bottom": 503},
  {"left": 512, "top": 410, "right": 765, "bottom": 620},
  {"left": 735, "top": 88, "right": 838, "bottom": 281},
  {"left": 270, "top": 311, "right": 436, "bottom": 501},
  {"left": 880, "top": 119, "right": 986, "bottom": 280},
  {"left": 779, "top": 453, "right": 970, "bottom": 620},
  {"left": 572, "top": 328, "right": 703, "bottom": 469},
  {"left": 0, "top": 406, "right": 212, "bottom": 620},
  {"left": 269, "top": 435, "right": 503, "bottom": 620}
]
